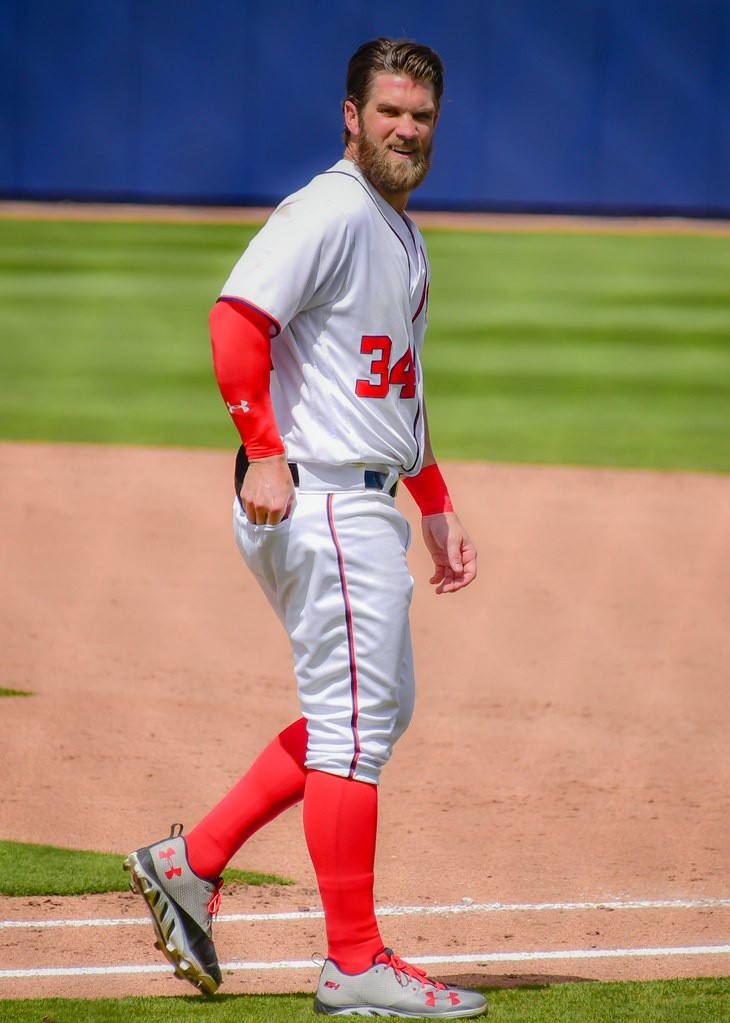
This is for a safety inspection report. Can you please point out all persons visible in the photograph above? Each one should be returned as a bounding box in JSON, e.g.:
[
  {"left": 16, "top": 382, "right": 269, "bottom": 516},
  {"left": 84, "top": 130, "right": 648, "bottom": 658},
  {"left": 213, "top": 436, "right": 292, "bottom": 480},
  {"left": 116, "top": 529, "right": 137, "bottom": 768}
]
[{"left": 123, "top": 40, "right": 488, "bottom": 1019}]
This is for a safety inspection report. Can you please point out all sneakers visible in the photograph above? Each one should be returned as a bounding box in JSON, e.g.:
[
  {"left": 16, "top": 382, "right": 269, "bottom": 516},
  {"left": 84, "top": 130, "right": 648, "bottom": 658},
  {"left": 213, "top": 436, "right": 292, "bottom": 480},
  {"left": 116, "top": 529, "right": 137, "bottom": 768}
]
[
  {"left": 311, "top": 947, "right": 489, "bottom": 1019},
  {"left": 123, "top": 823, "right": 225, "bottom": 997}
]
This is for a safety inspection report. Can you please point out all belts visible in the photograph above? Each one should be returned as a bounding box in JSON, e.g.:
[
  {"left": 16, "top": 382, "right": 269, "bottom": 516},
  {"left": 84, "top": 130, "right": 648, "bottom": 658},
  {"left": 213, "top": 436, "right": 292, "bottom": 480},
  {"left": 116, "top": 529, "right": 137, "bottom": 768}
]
[{"left": 288, "top": 462, "right": 398, "bottom": 497}]
